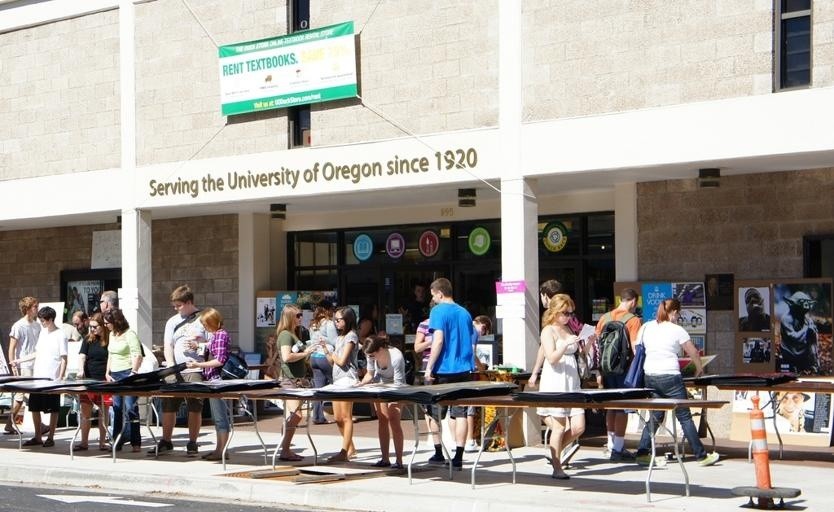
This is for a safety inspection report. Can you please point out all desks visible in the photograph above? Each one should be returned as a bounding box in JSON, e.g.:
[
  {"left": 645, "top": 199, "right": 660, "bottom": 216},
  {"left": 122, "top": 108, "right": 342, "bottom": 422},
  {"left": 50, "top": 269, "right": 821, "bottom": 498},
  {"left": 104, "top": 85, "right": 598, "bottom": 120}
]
[
  {"left": 219, "top": 389, "right": 454, "bottom": 487},
  {"left": 437, "top": 393, "right": 733, "bottom": 504},
  {"left": 716, "top": 375, "right": 834, "bottom": 462},
  {"left": 0, "top": 390, "right": 115, "bottom": 461},
  {"left": 104, "top": 391, "right": 269, "bottom": 472}
]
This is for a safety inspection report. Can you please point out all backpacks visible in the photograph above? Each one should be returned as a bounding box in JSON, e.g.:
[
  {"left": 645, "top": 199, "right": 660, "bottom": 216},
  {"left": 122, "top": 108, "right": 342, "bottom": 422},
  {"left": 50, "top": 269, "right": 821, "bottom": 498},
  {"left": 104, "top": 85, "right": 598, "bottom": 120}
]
[{"left": 599, "top": 311, "right": 636, "bottom": 377}]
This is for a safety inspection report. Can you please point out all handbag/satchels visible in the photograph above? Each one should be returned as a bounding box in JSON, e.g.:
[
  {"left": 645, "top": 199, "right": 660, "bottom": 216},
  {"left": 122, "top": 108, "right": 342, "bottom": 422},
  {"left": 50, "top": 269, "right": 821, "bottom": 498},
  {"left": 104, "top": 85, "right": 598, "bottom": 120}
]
[
  {"left": 220, "top": 353, "right": 249, "bottom": 380},
  {"left": 576, "top": 352, "right": 592, "bottom": 379},
  {"left": 131, "top": 343, "right": 159, "bottom": 374},
  {"left": 624, "top": 344, "right": 645, "bottom": 388}
]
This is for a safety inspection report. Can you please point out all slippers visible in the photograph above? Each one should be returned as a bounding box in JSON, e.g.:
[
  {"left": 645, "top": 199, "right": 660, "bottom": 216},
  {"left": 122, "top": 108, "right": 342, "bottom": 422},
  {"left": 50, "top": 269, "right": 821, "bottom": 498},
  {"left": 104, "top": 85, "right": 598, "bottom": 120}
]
[{"left": 278, "top": 455, "right": 462, "bottom": 472}]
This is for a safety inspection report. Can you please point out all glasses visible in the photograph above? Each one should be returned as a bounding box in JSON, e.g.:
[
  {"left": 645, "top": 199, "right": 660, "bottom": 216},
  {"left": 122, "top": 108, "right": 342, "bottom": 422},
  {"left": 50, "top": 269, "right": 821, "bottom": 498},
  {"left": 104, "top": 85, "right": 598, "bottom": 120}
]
[
  {"left": 297, "top": 313, "right": 302, "bottom": 318},
  {"left": 562, "top": 312, "right": 577, "bottom": 317},
  {"left": 335, "top": 317, "right": 343, "bottom": 323},
  {"left": 89, "top": 324, "right": 99, "bottom": 328}
]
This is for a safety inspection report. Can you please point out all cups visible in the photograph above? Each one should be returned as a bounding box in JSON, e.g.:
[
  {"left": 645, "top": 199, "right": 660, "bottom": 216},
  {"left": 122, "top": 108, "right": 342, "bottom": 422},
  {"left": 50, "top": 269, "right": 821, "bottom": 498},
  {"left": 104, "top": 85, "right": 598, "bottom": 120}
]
[{"left": 664, "top": 452, "right": 674, "bottom": 461}]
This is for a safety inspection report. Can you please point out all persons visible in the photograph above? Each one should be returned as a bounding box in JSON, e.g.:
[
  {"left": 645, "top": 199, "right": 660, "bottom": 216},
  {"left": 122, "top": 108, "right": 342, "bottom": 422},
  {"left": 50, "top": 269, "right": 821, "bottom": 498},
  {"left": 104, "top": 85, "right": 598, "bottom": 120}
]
[
  {"left": 635, "top": 298, "right": 719, "bottom": 467},
  {"left": 535, "top": 293, "right": 597, "bottom": 479},
  {"left": 309, "top": 299, "right": 360, "bottom": 425},
  {"left": 275, "top": 303, "right": 319, "bottom": 461},
  {"left": 65, "top": 290, "right": 121, "bottom": 452},
  {"left": 146, "top": 284, "right": 211, "bottom": 454},
  {"left": 448, "top": 315, "right": 493, "bottom": 453},
  {"left": 739, "top": 288, "right": 770, "bottom": 331},
  {"left": 355, "top": 279, "right": 492, "bottom": 386},
  {"left": 8, "top": 305, "right": 67, "bottom": 447},
  {"left": 676, "top": 316, "right": 703, "bottom": 331},
  {"left": 776, "top": 391, "right": 815, "bottom": 433},
  {"left": 527, "top": 279, "right": 582, "bottom": 471},
  {"left": 317, "top": 306, "right": 360, "bottom": 464},
  {"left": 7, "top": 296, "right": 42, "bottom": 435},
  {"left": 595, "top": 288, "right": 642, "bottom": 462},
  {"left": 262, "top": 334, "right": 282, "bottom": 380},
  {"left": 423, "top": 276, "right": 472, "bottom": 471},
  {"left": 101, "top": 308, "right": 143, "bottom": 453},
  {"left": 186, "top": 306, "right": 231, "bottom": 461},
  {"left": 351, "top": 330, "right": 406, "bottom": 469}
]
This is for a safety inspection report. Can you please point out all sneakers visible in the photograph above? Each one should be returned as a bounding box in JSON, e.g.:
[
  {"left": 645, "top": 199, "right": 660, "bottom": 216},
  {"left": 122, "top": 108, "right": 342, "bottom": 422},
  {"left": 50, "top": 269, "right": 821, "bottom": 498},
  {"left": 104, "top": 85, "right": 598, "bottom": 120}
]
[
  {"left": 545, "top": 446, "right": 720, "bottom": 479},
  {"left": 21, "top": 437, "right": 228, "bottom": 460}
]
